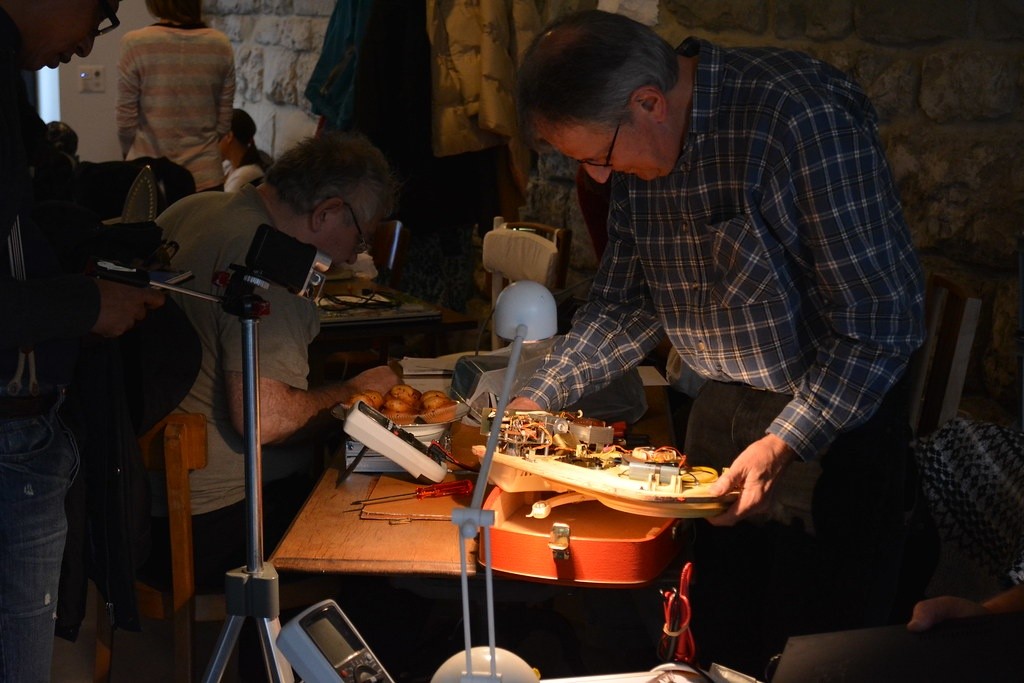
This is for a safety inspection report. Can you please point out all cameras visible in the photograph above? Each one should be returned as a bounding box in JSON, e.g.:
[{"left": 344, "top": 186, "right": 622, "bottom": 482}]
[{"left": 244, "top": 223, "right": 332, "bottom": 305}]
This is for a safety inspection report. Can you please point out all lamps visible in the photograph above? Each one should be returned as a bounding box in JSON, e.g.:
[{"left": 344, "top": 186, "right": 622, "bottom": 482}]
[{"left": 450, "top": 279, "right": 562, "bottom": 683}]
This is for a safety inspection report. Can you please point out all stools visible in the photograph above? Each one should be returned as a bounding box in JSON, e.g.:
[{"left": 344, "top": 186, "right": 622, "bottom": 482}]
[{"left": 608, "top": 364, "right": 680, "bottom": 453}]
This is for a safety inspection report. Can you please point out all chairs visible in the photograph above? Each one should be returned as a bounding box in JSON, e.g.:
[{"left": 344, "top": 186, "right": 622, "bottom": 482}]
[{"left": 435, "top": 216, "right": 572, "bottom": 360}]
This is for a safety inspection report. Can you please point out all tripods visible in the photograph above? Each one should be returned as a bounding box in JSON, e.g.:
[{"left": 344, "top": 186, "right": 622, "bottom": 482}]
[{"left": 145, "top": 278, "right": 296, "bottom": 683}]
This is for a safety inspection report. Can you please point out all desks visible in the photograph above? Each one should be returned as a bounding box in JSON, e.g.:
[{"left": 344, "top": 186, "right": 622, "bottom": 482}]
[
  {"left": 268, "top": 414, "right": 498, "bottom": 663},
  {"left": 312, "top": 279, "right": 443, "bottom": 368}
]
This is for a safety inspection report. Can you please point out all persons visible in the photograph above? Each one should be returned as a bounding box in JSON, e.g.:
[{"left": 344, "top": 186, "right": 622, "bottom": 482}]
[
  {"left": 487, "top": 9, "right": 934, "bottom": 671},
  {"left": 217, "top": 109, "right": 278, "bottom": 199},
  {"left": 908, "top": 561, "right": 1023, "bottom": 636},
  {"left": 137, "top": 128, "right": 397, "bottom": 584},
  {"left": 115, "top": 1, "right": 241, "bottom": 193},
  {"left": 1, "top": 1, "right": 150, "bottom": 681}
]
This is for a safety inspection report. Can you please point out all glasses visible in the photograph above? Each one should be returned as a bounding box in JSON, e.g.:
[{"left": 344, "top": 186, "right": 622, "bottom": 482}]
[
  {"left": 576, "top": 121, "right": 622, "bottom": 168},
  {"left": 91, "top": 0, "right": 121, "bottom": 38},
  {"left": 324, "top": 196, "right": 370, "bottom": 256}
]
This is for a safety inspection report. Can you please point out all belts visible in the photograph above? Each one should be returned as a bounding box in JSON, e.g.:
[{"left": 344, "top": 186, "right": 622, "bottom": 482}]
[{"left": 0, "top": 383, "right": 67, "bottom": 426}]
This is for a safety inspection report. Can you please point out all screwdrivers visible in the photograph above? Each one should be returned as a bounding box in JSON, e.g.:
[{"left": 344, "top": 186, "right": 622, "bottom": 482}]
[{"left": 349, "top": 480, "right": 472, "bottom": 506}]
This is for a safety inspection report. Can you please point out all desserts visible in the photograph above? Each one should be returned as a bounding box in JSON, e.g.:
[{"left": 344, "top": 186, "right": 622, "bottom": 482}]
[{"left": 340, "top": 384, "right": 460, "bottom": 425}]
